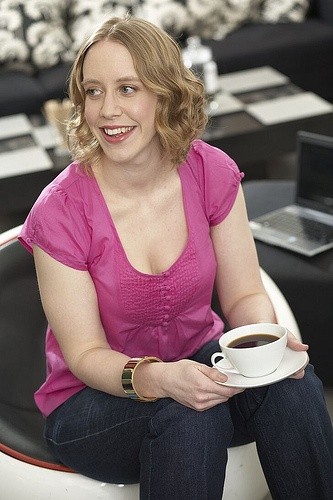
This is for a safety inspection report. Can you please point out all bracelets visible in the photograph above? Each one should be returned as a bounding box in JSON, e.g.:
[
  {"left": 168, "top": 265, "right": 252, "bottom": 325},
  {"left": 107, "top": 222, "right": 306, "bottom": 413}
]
[{"left": 121, "top": 355, "right": 163, "bottom": 403}]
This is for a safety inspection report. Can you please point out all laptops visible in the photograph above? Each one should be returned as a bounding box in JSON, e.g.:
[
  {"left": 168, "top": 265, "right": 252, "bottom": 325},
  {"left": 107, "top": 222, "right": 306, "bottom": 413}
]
[{"left": 249, "top": 131, "right": 333, "bottom": 256}]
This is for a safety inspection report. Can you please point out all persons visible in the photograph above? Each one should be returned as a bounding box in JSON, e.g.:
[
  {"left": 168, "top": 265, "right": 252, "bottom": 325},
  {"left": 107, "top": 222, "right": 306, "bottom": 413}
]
[{"left": 17, "top": 17, "right": 333, "bottom": 500}]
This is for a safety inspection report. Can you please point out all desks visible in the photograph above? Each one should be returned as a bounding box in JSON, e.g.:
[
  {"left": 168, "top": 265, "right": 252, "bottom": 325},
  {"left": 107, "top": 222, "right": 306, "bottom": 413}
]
[
  {"left": 1, "top": 63, "right": 332, "bottom": 218},
  {"left": 241, "top": 178, "right": 332, "bottom": 359}
]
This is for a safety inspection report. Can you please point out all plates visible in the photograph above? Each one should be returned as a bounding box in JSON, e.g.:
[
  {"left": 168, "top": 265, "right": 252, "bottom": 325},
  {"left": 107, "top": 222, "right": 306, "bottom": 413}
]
[{"left": 211, "top": 349, "right": 308, "bottom": 387}]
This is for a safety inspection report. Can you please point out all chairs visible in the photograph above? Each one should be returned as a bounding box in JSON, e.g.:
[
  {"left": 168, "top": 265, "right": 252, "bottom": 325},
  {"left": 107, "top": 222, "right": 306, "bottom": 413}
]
[{"left": 0, "top": 224, "right": 303, "bottom": 499}]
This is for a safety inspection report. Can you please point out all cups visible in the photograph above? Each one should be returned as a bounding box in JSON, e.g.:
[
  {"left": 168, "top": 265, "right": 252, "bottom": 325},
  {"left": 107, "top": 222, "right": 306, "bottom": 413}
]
[{"left": 211, "top": 323, "right": 287, "bottom": 378}]
